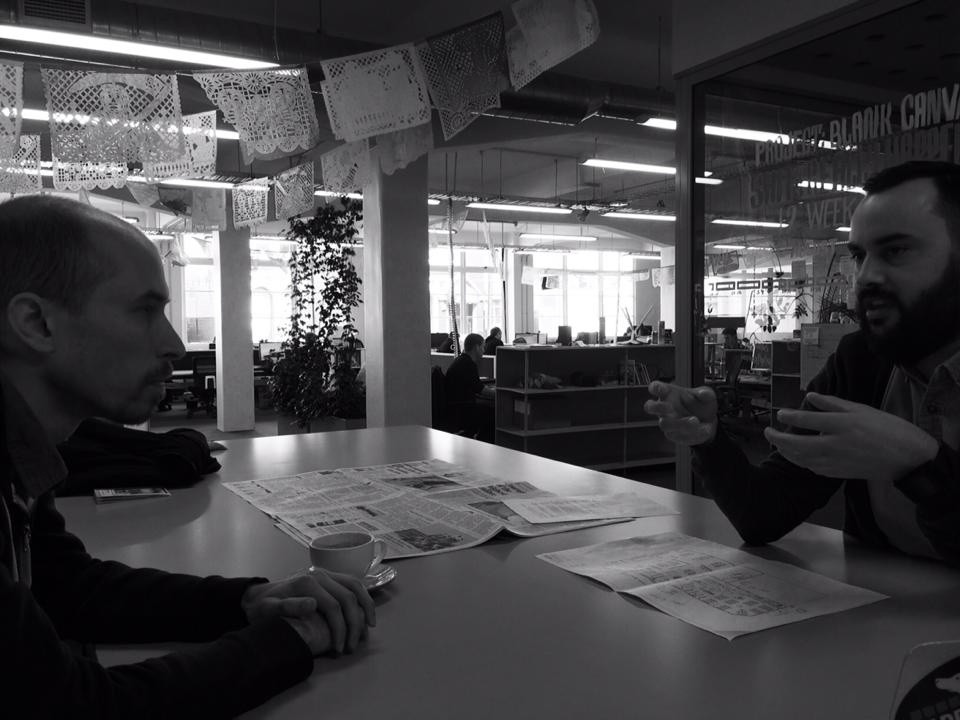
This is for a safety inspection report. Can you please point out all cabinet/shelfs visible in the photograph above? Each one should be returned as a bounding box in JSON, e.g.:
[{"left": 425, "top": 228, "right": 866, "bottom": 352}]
[{"left": 495, "top": 345, "right": 676, "bottom": 469}]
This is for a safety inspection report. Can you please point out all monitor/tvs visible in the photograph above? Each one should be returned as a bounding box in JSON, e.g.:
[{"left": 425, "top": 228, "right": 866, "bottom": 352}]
[{"left": 749, "top": 342, "right": 771, "bottom": 376}]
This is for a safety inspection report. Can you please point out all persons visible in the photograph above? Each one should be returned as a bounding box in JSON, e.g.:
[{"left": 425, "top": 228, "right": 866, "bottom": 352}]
[
  {"left": 721, "top": 327, "right": 747, "bottom": 350},
  {"left": 484, "top": 328, "right": 503, "bottom": 355},
  {"left": 0, "top": 196, "right": 377, "bottom": 720},
  {"left": 295, "top": 333, "right": 328, "bottom": 373},
  {"left": 445, "top": 334, "right": 496, "bottom": 441},
  {"left": 643, "top": 161, "right": 960, "bottom": 571}
]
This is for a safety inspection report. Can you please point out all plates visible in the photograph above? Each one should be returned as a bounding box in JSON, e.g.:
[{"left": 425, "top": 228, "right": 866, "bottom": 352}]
[{"left": 307, "top": 557, "right": 398, "bottom": 594}]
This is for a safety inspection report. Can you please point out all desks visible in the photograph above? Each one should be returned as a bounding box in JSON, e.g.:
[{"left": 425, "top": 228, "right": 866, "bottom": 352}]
[{"left": 51, "top": 427, "right": 960, "bottom": 720}]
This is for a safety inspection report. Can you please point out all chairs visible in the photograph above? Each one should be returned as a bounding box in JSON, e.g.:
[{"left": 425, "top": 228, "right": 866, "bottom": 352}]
[{"left": 431, "top": 365, "right": 494, "bottom": 443}]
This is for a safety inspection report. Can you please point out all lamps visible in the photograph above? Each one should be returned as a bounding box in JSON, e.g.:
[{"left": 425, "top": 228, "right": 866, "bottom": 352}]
[
  {"left": 464, "top": 151, "right": 573, "bottom": 214},
  {"left": 577, "top": 137, "right": 677, "bottom": 175},
  {"left": 636, "top": 17, "right": 858, "bottom": 152}
]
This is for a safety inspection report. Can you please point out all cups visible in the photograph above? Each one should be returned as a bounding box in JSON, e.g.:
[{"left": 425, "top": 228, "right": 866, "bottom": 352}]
[{"left": 309, "top": 530, "right": 387, "bottom": 583}]
